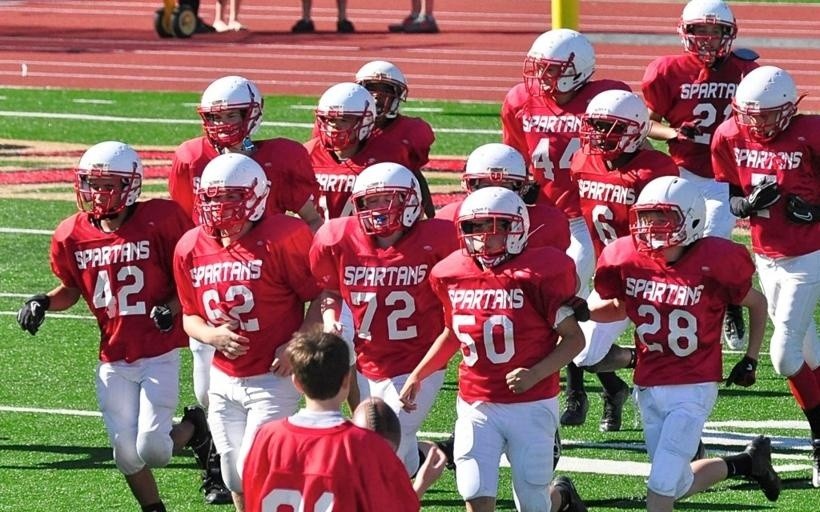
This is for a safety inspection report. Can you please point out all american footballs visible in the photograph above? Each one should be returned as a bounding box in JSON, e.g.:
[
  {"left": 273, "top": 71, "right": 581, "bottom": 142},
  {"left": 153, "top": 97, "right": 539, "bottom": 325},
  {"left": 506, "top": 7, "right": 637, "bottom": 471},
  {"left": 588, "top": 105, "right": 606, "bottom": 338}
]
[{"left": 353, "top": 397, "right": 399, "bottom": 451}]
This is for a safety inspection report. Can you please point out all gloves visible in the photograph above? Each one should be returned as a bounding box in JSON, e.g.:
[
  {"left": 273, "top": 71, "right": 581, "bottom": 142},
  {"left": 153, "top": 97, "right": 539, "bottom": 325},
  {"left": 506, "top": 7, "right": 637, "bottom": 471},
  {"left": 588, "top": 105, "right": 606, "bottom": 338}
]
[
  {"left": 17, "top": 293, "right": 51, "bottom": 336},
  {"left": 783, "top": 190, "right": 816, "bottom": 226},
  {"left": 673, "top": 117, "right": 704, "bottom": 146},
  {"left": 562, "top": 294, "right": 591, "bottom": 324},
  {"left": 519, "top": 181, "right": 543, "bottom": 205},
  {"left": 732, "top": 176, "right": 781, "bottom": 218},
  {"left": 149, "top": 302, "right": 177, "bottom": 335},
  {"left": 724, "top": 355, "right": 758, "bottom": 389}
]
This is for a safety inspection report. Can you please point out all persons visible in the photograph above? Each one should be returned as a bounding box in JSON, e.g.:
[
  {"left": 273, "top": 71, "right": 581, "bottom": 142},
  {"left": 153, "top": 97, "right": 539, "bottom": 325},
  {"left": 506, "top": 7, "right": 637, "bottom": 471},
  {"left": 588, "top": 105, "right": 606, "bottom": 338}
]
[
  {"left": 12, "top": 136, "right": 234, "bottom": 511},
  {"left": 352, "top": 59, "right": 437, "bottom": 223},
  {"left": 289, "top": 0, "right": 355, "bottom": 35},
  {"left": 168, "top": 153, "right": 328, "bottom": 511},
  {"left": 303, "top": 160, "right": 567, "bottom": 483},
  {"left": 163, "top": 68, "right": 327, "bottom": 504},
  {"left": 232, "top": 327, "right": 451, "bottom": 510},
  {"left": 212, "top": 1, "right": 254, "bottom": 36},
  {"left": 179, "top": 1, "right": 219, "bottom": 40},
  {"left": 297, "top": 79, "right": 419, "bottom": 417}
]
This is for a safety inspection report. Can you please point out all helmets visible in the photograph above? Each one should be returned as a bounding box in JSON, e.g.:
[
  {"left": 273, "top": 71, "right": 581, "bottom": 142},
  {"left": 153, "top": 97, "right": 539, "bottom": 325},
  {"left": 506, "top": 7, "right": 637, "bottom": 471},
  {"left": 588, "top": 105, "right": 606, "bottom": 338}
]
[
  {"left": 197, "top": 75, "right": 266, "bottom": 147},
  {"left": 354, "top": 59, "right": 409, "bottom": 122},
  {"left": 315, "top": 80, "right": 374, "bottom": 152},
  {"left": 522, "top": 28, "right": 598, "bottom": 99},
  {"left": 74, "top": 140, "right": 143, "bottom": 219},
  {"left": 191, "top": 153, "right": 269, "bottom": 240},
  {"left": 464, "top": 142, "right": 525, "bottom": 189},
  {"left": 678, "top": 0, "right": 737, "bottom": 62},
  {"left": 633, "top": 176, "right": 708, "bottom": 253},
  {"left": 580, "top": 89, "right": 651, "bottom": 159},
  {"left": 351, "top": 161, "right": 422, "bottom": 235},
  {"left": 730, "top": 65, "right": 800, "bottom": 143},
  {"left": 452, "top": 186, "right": 530, "bottom": 267}
]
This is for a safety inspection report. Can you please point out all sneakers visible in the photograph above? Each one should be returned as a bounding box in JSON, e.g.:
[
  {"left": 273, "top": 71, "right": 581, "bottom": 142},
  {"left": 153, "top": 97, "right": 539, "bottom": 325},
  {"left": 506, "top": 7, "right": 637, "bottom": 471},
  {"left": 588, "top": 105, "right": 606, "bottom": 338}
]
[
  {"left": 552, "top": 475, "right": 589, "bottom": 512},
  {"left": 291, "top": 17, "right": 316, "bottom": 33},
  {"left": 561, "top": 389, "right": 591, "bottom": 426},
  {"left": 336, "top": 16, "right": 357, "bottom": 34},
  {"left": 388, "top": 12, "right": 442, "bottom": 34},
  {"left": 183, "top": 402, "right": 235, "bottom": 505},
  {"left": 721, "top": 301, "right": 750, "bottom": 350},
  {"left": 743, "top": 436, "right": 783, "bottom": 502},
  {"left": 598, "top": 378, "right": 630, "bottom": 434},
  {"left": 212, "top": 16, "right": 251, "bottom": 35}
]
